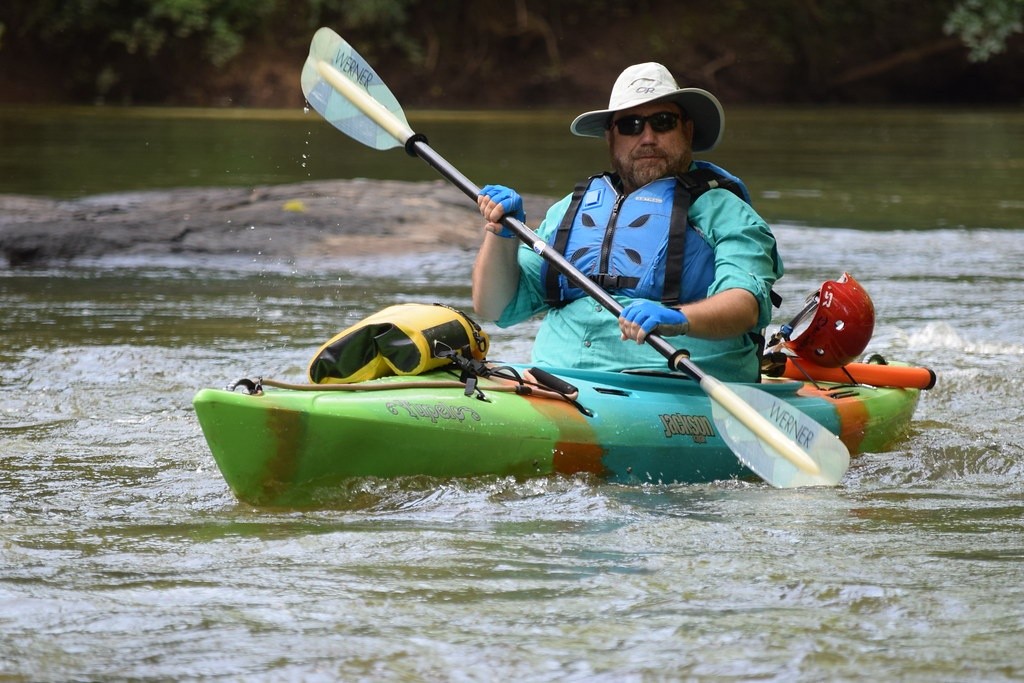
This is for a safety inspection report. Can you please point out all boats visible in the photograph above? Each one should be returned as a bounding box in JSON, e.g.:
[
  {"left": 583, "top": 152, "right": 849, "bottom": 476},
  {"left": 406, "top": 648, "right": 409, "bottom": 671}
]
[{"left": 195, "top": 357, "right": 925, "bottom": 504}]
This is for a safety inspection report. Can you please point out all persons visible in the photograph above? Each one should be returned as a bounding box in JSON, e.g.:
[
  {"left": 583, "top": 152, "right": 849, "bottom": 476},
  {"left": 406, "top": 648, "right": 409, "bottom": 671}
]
[{"left": 473, "top": 60, "right": 782, "bottom": 384}]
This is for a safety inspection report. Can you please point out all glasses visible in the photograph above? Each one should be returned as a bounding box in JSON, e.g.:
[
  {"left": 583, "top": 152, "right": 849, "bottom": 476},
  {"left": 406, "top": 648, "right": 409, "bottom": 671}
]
[{"left": 610, "top": 111, "right": 681, "bottom": 135}]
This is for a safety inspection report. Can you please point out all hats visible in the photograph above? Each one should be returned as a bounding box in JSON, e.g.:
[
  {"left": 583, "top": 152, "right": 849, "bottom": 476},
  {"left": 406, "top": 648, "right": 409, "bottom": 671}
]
[{"left": 569, "top": 62, "right": 725, "bottom": 154}]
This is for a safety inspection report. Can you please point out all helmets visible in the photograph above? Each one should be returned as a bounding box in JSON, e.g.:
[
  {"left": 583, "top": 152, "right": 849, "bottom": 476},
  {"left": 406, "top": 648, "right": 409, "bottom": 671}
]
[{"left": 784, "top": 272, "right": 875, "bottom": 368}]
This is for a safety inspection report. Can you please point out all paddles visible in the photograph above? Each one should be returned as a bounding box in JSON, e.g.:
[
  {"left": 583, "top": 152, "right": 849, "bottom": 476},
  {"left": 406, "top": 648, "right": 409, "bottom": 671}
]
[{"left": 300, "top": 25, "right": 853, "bottom": 488}]
